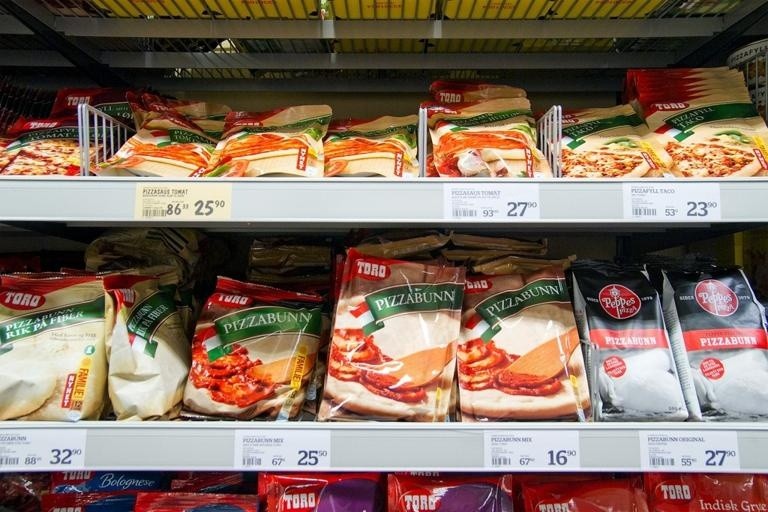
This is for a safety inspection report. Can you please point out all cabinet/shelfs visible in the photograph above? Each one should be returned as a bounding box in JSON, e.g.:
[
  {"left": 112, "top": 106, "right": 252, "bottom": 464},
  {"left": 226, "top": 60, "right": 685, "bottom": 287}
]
[{"left": 0, "top": 0, "right": 768, "bottom": 512}]
